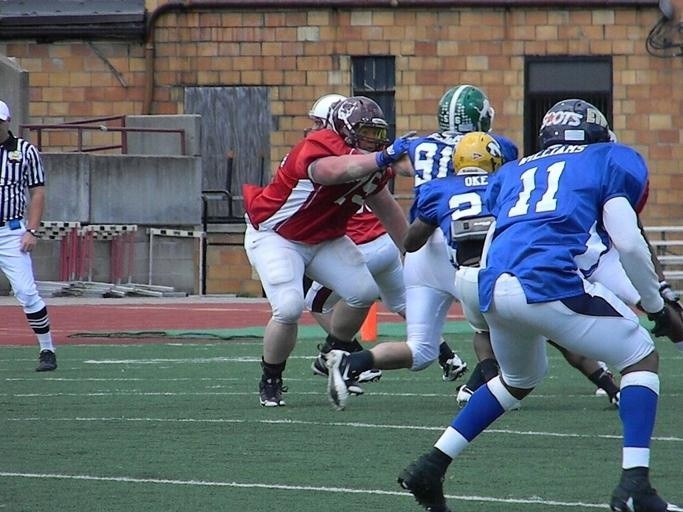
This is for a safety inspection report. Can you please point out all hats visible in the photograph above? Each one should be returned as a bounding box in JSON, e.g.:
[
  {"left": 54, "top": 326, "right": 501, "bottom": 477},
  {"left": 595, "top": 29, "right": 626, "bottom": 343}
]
[{"left": 0, "top": 100, "right": 11, "bottom": 120}]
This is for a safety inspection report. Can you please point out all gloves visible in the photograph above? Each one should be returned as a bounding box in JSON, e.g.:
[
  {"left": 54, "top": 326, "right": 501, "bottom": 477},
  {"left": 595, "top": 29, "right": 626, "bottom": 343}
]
[{"left": 636, "top": 293, "right": 683, "bottom": 343}]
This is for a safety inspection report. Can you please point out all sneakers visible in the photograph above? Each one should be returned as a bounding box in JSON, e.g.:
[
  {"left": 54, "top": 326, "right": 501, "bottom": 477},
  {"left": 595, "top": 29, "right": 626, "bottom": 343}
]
[
  {"left": 36, "top": 349, "right": 57, "bottom": 372},
  {"left": 397, "top": 455, "right": 451, "bottom": 512},
  {"left": 260, "top": 342, "right": 476, "bottom": 411},
  {"left": 611, "top": 479, "right": 682, "bottom": 512}
]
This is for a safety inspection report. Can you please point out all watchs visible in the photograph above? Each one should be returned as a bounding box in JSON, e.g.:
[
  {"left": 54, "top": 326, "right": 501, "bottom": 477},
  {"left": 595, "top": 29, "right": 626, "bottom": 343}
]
[{"left": 26, "top": 227, "right": 38, "bottom": 235}]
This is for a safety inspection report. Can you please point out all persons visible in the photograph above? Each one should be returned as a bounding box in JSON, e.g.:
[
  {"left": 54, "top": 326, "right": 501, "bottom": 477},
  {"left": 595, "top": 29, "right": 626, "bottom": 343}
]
[
  {"left": 0, "top": 97, "right": 58, "bottom": 373},
  {"left": 323, "top": 83, "right": 519, "bottom": 424},
  {"left": 303, "top": 92, "right": 467, "bottom": 384},
  {"left": 239, "top": 93, "right": 418, "bottom": 407},
  {"left": 405, "top": 129, "right": 620, "bottom": 414},
  {"left": 395, "top": 95, "right": 682, "bottom": 510}
]
